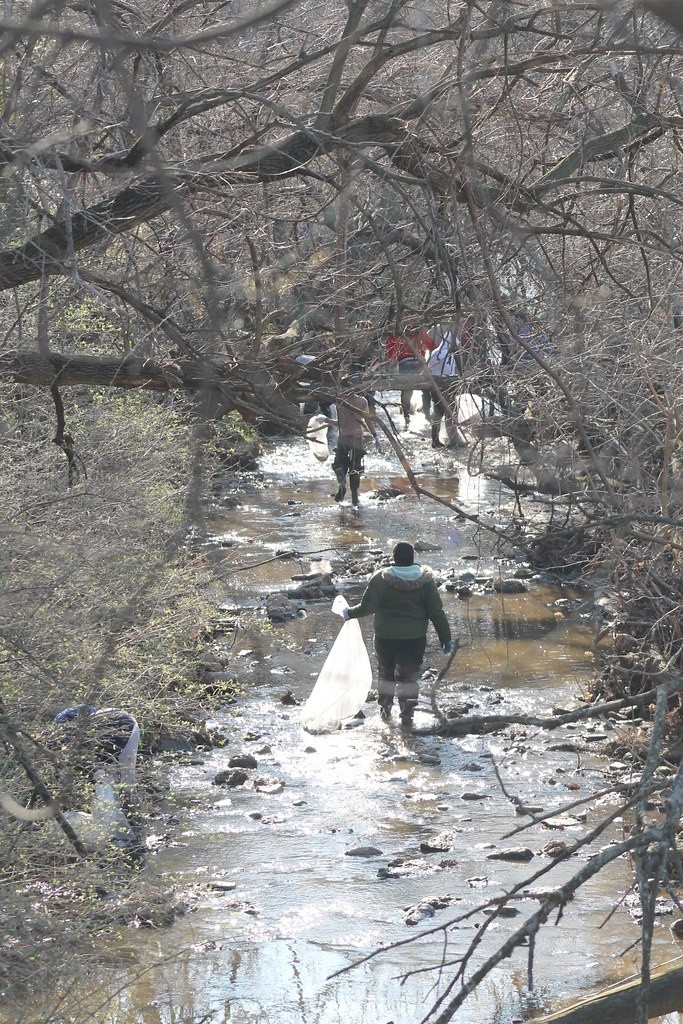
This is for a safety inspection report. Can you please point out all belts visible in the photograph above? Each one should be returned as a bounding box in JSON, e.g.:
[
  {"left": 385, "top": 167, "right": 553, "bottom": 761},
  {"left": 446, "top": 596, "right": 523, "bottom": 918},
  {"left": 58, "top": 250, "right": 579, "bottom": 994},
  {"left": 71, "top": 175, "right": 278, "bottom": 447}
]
[{"left": 398, "top": 356, "right": 425, "bottom": 364}]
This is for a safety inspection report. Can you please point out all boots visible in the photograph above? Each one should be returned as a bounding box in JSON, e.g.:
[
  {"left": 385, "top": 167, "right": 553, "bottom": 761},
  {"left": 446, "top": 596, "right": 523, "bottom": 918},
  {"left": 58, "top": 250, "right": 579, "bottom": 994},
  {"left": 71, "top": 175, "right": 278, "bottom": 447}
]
[
  {"left": 378, "top": 699, "right": 393, "bottom": 719},
  {"left": 397, "top": 701, "right": 418, "bottom": 726},
  {"left": 424, "top": 408, "right": 431, "bottom": 423},
  {"left": 403, "top": 413, "right": 411, "bottom": 431},
  {"left": 432, "top": 420, "right": 445, "bottom": 448},
  {"left": 350, "top": 473, "right": 360, "bottom": 506},
  {"left": 335, "top": 467, "right": 348, "bottom": 502},
  {"left": 447, "top": 421, "right": 470, "bottom": 447}
]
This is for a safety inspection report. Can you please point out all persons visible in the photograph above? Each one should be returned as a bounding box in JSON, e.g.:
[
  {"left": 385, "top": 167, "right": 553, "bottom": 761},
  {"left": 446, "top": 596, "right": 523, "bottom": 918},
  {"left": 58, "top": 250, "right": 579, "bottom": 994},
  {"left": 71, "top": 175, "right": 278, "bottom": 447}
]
[
  {"left": 343, "top": 541, "right": 453, "bottom": 728},
  {"left": 302, "top": 314, "right": 553, "bottom": 508}
]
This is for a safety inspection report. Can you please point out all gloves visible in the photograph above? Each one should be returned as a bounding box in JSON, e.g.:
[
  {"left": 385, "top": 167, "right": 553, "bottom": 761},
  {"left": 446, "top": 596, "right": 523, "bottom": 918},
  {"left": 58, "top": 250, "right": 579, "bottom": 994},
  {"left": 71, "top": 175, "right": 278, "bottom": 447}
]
[
  {"left": 441, "top": 640, "right": 451, "bottom": 654},
  {"left": 343, "top": 607, "right": 350, "bottom": 621},
  {"left": 315, "top": 414, "right": 327, "bottom": 424},
  {"left": 376, "top": 438, "right": 381, "bottom": 453}
]
